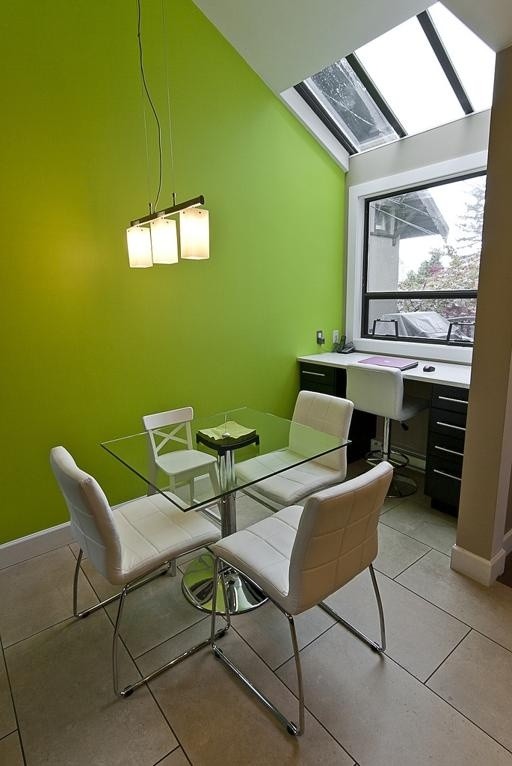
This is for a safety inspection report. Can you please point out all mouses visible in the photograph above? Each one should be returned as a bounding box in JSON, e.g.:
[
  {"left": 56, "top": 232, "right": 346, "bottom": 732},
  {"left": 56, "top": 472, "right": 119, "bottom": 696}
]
[{"left": 423, "top": 364, "right": 435, "bottom": 372}]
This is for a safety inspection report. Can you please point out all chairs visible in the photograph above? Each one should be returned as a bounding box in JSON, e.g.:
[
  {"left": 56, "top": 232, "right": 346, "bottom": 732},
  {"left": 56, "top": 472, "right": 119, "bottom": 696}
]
[
  {"left": 142, "top": 406, "right": 220, "bottom": 508},
  {"left": 232, "top": 390, "right": 354, "bottom": 513},
  {"left": 345, "top": 364, "right": 419, "bottom": 497},
  {"left": 48, "top": 446, "right": 230, "bottom": 698},
  {"left": 210, "top": 460, "right": 394, "bottom": 737}
]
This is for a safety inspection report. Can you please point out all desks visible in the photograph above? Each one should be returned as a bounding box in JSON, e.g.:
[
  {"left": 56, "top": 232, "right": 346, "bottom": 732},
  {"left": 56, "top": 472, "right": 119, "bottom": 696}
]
[
  {"left": 296, "top": 351, "right": 472, "bottom": 518},
  {"left": 100, "top": 407, "right": 351, "bottom": 615}
]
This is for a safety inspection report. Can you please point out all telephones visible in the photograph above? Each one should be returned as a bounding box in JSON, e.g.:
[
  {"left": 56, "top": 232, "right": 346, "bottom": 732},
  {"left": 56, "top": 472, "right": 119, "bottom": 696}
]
[{"left": 336, "top": 335, "right": 355, "bottom": 353}]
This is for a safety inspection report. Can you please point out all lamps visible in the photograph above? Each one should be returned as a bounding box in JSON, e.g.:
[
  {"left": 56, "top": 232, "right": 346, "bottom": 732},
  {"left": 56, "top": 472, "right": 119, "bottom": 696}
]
[{"left": 126, "top": 0, "right": 209, "bottom": 267}]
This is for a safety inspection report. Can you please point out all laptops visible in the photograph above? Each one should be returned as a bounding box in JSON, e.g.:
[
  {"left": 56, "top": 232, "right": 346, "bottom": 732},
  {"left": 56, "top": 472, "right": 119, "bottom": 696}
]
[{"left": 358, "top": 354, "right": 418, "bottom": 371}]
